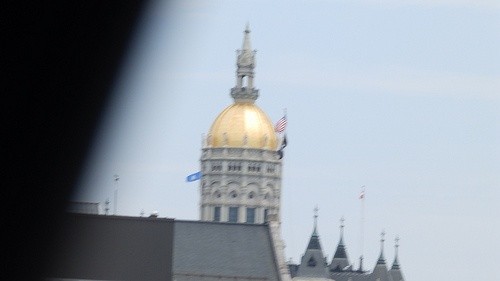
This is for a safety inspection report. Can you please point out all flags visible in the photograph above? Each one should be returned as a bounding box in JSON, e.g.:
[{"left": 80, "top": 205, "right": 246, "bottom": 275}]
[
  {"left": 359, "top": 190, "right": 365, "bottom": 199},
  {"left": 186, "top": 169, "right": 204, "bottom": 182},
  {"left": 273, "top": 115, "right": 287, "bottom": 133},
  {"left": 276, "top": 134, "right": 287, "bottom": 160}
]
